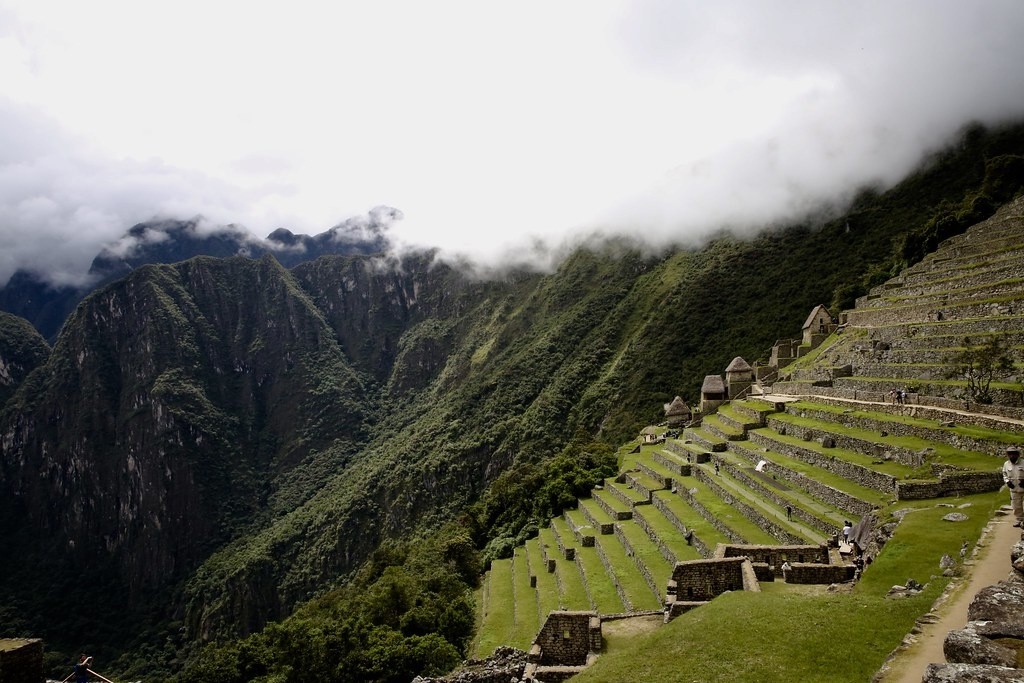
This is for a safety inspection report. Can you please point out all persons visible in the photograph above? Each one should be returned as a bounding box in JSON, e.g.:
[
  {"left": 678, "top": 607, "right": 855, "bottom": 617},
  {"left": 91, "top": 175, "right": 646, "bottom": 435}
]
[
  {"left": 843, "top": 519, "right": 852, "bottom": 543},
  {"left": 1002, "top": 446, "right": 1024, "bottom": 529},
  {"left": 686, "top": 451, "right": 691, "bottom": 463},
  {"left": 889, "top": 388, "right": 907, "bottom": 405},
  {"left": 714, "top": 463, "right": 719, "bottom": 475},
  {"left": 787, "top": 506, "right": 791, "bottom": 521}
]
